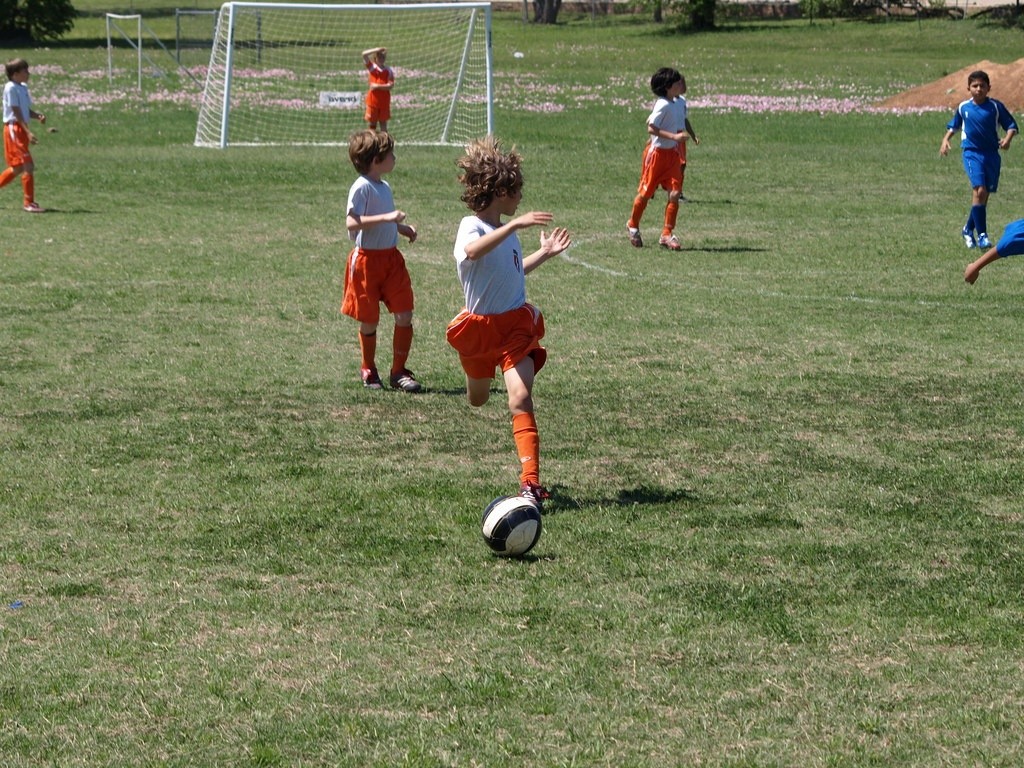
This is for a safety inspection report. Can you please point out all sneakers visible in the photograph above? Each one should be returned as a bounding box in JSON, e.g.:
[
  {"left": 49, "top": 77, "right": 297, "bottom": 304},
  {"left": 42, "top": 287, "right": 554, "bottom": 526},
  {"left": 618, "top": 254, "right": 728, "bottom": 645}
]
[
  {"left": 659, "top": 233, "right": 680, "bottom": 251},
  {"left": 391, "top": 368, "right": 421, "bottom": 392},
  {"left": 518, "top": 477, "right": 542, "bottom": 514},
  {"left": 626, "top": 219, "right": 643, "bottom": 248},
  {"left": 978, "top": 231, "right": 992, "bottom": 249},
  {"left": 963, "top": 225, "right": 977, "bottom": 248},
  {"left": 361, "top": 363, "right": 384, "bottom": 389}
]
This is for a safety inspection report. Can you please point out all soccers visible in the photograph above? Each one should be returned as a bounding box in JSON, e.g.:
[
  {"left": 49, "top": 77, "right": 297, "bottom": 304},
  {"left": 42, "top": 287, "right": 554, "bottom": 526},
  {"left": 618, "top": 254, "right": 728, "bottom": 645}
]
[{"left": 481, "top": 496, "right": 542, "bottom": 557}]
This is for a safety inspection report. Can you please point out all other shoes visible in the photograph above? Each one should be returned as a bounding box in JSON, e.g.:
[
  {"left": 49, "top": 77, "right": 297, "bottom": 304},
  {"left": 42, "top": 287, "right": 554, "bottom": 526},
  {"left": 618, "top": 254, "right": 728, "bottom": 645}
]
[{"left": 23, "top": 202, "right": 46, "bottom": 212}]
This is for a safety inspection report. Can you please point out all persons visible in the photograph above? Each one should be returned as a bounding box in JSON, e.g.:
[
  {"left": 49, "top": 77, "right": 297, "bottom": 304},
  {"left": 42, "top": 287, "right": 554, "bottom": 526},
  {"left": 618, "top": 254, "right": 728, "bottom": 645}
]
[
  {"left": 964, "top": 219, "right": 1024, "bottom": 284},
  {"left": 940, "top": 71, "right": 1018, "bottom": 250},
  {"left": 446, "top": 137, "right": 573, "bottom": 512},
  {"left": 625, "top": 68, "right": 700, "bottom": 249},
  {"left": 0, "top": 58, "right": 47, "bottom": 212},
  {"left": 362, "top": 47, "right": 394, "bottom": 132},
  {"left": 340, "top": 129, "right": 421, "bottom": 391}
]
